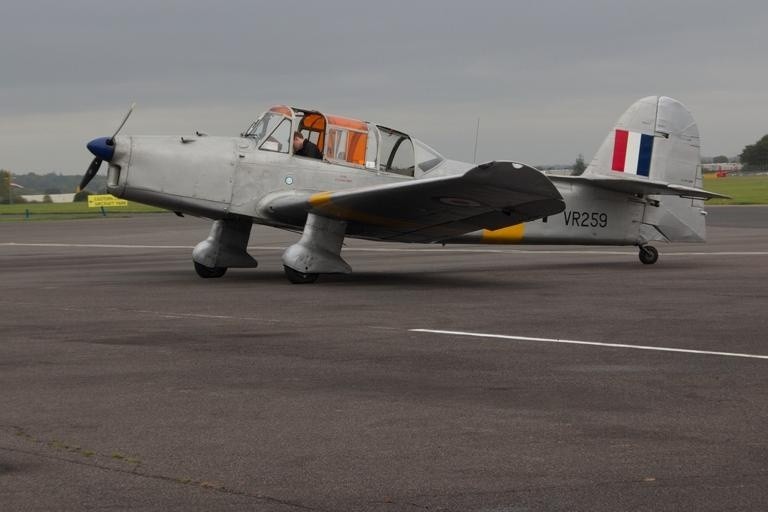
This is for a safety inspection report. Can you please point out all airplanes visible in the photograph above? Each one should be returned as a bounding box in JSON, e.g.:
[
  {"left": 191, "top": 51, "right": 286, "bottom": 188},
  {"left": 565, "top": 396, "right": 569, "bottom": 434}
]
[{"left": 71, "top": 89, "right": 729, "bottom": 278}]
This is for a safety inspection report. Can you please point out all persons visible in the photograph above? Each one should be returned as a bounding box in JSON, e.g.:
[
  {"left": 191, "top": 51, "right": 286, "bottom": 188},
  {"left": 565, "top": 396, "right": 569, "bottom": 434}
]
[{"left": 288, "top": 130, "right": 323, "bottom": 160}]
